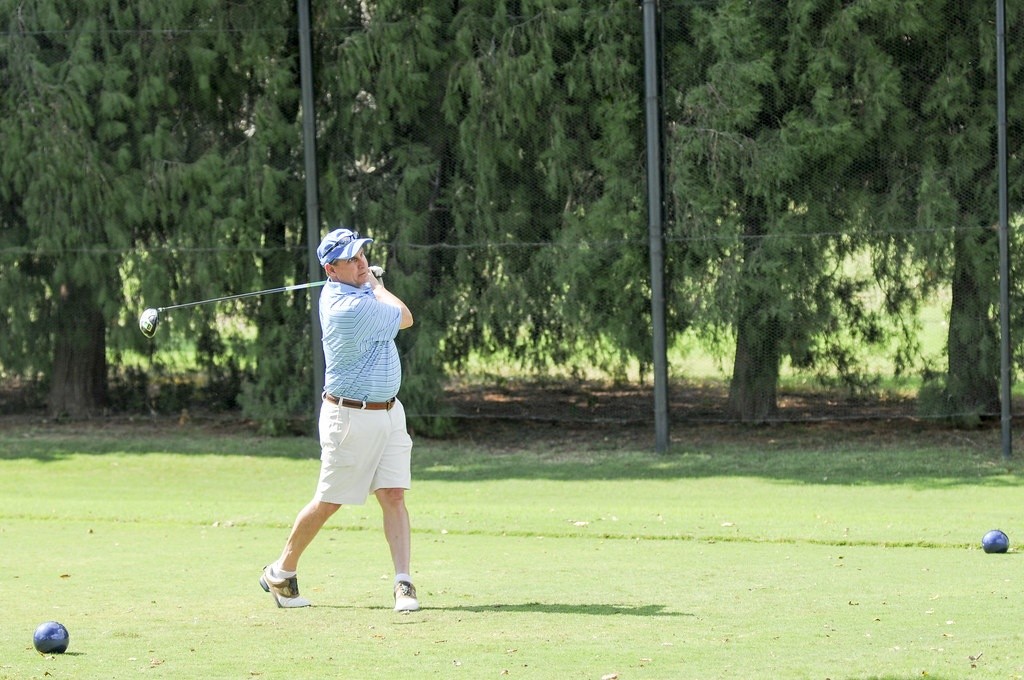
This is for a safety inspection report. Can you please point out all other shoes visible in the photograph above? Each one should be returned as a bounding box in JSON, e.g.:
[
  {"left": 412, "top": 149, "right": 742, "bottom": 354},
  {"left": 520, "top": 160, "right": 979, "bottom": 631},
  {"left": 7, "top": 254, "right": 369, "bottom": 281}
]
[
  {"left": 392, "top": 580, "right": 419, "bottom": 611},
  {"left": 258, "top": 565, "right": 312, "bottom": 608}
]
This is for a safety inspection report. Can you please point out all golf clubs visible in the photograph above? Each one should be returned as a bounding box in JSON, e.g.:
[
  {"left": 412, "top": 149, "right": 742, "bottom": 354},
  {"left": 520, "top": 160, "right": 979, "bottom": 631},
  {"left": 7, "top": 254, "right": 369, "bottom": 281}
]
[{"left": 139, "top": 271, "right": 388, "bottom": 339}]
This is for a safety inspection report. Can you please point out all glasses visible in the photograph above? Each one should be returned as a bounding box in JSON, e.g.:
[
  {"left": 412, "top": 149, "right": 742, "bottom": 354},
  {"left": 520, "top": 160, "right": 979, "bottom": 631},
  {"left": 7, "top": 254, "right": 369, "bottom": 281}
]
[{"left": 322, "top": 231, "right": 360, "bottom": 257}]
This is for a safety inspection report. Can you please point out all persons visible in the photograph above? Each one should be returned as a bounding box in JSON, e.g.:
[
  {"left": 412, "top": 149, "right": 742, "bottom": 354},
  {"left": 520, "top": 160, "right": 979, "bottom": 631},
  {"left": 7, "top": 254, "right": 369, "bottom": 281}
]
[{"left": 259, "top": 228, "right": 417, "bottom": 610}]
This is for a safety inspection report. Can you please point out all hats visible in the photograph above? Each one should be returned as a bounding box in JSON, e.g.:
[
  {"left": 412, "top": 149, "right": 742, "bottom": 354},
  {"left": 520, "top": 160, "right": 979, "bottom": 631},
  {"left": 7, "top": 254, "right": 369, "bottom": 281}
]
[{"left": 316, "top": 228, "right": 374, "bottom": 265}]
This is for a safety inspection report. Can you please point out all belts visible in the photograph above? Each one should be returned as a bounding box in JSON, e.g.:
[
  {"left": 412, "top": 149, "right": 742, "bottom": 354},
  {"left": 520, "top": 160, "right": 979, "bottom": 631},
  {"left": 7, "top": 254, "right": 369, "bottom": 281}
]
[{"left": 324, "top": 391, "right": 396, "bottom": 410}]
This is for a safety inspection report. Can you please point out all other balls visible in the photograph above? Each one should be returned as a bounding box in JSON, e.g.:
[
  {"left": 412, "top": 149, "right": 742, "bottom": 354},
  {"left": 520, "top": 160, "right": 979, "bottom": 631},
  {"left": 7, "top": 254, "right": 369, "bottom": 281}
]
[
  {"left": 33, "top": 620, "right": 71, "bottom": 656},
  {"left": 982, "top": 530, "right": 1010, "bottom": 554}
]
